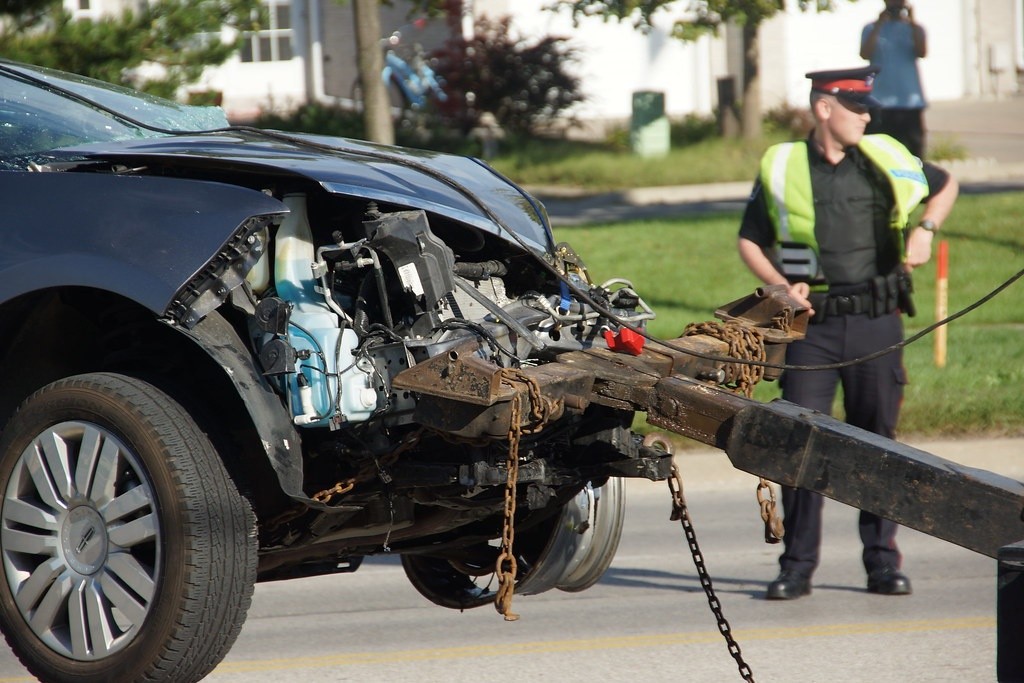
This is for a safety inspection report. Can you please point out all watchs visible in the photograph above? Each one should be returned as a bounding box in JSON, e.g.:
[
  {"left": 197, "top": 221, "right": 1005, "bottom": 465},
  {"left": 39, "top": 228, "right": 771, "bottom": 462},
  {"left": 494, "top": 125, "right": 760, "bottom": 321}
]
[{"left": 919, "top": 220, "right": 936, "bottom": 236}]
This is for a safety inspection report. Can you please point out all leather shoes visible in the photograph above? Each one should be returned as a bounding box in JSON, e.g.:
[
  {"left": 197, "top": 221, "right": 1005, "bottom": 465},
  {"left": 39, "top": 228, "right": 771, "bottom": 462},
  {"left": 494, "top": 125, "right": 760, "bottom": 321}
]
[
  {"left": 867, "top": 566, "right": 910, "bottom": 594},
  {"left": 765, "top": 570, "right": 810, "bottom": 599}
]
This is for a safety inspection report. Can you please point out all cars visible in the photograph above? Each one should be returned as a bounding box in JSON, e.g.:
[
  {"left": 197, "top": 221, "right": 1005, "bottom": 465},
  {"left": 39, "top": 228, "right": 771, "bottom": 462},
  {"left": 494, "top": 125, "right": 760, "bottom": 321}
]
[{"left": 1, "top": 58, "right": 624, "bottom": 683}]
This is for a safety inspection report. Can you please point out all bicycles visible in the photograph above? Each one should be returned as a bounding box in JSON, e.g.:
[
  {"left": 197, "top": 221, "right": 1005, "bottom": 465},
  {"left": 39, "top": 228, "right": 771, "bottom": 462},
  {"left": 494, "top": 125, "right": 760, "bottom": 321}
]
[{"left": 349, "top": 31, "right": 455, "bottom": 129}]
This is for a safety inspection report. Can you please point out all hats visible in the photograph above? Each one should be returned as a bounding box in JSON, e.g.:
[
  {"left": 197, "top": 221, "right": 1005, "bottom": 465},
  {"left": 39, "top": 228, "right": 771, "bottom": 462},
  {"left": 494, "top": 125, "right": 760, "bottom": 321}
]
[{"left": 806, "top": 66, "right": 882, "bottom": 108}]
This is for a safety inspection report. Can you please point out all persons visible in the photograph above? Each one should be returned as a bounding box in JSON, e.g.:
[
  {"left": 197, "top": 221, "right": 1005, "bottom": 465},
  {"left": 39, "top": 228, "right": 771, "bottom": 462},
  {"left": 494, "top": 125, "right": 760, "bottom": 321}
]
[
  {"left": 860, "top": 0, "right": 926, "bottom": 157},
  {"left": 738, "top": 66, "right": 958, "bottom": 599}
]
